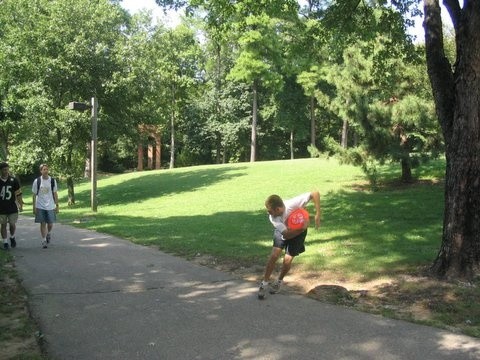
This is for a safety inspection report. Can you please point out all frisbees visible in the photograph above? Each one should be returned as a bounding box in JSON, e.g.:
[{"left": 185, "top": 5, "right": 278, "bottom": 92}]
[{"left": 287, "top": 208, "right": 310, "bottom": 230}]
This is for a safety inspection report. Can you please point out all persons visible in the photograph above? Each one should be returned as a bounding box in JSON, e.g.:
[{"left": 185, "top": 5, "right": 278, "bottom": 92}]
[
  {"left": 257, "top": 190, "right": 321, "bottom": 298},
  {"left": 0, "top": 162, "right": 23, "bottom": 249},
  {"left": 32, "top": 164, "right": 59, "bottom": 248}
]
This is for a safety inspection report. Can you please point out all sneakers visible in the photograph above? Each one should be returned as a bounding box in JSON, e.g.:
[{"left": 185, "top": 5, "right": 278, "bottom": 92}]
[
  {"left": 10, "top": 237, "right": 16, "bottom": 248},
  {"left": 46, "top": 234, "right": 51, "bottom": 243},
  {"left": 41, "top": 241, "right": 47, "bottom": 248},
  {"left": 258, "top": 282, "right": 271, "bottom": 299},
  {"left": 269, "top": 282, "right": 281, "bottom": 294},
  {"left": 4, "top": 243, "right": 8, "bottom": 248}
]
